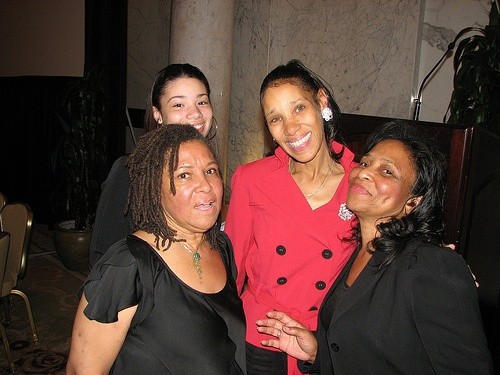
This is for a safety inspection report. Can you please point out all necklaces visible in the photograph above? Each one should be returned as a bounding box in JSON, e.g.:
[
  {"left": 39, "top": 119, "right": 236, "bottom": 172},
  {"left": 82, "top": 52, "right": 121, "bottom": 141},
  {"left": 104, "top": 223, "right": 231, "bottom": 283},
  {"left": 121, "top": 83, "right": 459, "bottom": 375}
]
[
  {"left": 169, "top": 233, "right": 211, "bottom": 276},
  {"left": 286, "top": 158, "right": 337, "bottom": 202}
]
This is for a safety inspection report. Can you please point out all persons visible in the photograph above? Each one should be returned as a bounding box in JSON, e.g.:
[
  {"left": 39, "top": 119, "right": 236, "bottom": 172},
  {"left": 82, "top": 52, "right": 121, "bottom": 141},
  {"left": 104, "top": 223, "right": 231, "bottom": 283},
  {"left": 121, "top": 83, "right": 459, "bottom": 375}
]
[
  {"left": 66, "top": 124, "right": 247, "bottom": 374},
  {"left": 89, "top": 63, "right": 213, "bottom": 274},
  {"left": 223, "top": 59, "right": 361, "bottom": 374},
  {"left": 255, "top": 121, "right": 500, "bottom": 375}
]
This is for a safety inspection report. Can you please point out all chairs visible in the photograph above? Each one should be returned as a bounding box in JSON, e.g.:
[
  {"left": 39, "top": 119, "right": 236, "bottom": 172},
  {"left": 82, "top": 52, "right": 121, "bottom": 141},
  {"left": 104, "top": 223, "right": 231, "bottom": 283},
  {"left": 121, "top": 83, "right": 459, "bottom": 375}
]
[
  {"left": 0, "top": 193, "right": 5, "bottom": 213},
  {"left": 0, "top": 231, "right": 16, "bottom": 375},
  {"left": 0, "top": 202, "right": 39, "bottom": 343}
]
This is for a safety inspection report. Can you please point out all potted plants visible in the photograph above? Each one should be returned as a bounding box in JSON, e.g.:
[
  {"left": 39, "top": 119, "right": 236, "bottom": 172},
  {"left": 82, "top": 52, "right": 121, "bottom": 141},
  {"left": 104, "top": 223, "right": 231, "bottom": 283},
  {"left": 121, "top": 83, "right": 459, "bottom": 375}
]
[{"left": 53, "top": 71, "right": 108, "bottom": 272}]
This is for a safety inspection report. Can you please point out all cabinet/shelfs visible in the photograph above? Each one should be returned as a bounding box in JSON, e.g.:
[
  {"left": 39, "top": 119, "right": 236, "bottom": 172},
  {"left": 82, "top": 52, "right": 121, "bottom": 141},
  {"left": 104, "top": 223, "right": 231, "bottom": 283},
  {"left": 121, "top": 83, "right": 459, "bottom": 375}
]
[{"left": 327, "top": 112, "right": 500, "bottom": 306}]
